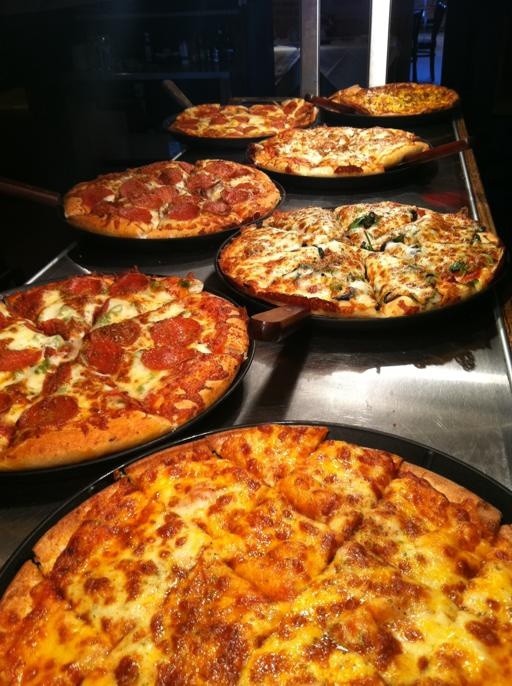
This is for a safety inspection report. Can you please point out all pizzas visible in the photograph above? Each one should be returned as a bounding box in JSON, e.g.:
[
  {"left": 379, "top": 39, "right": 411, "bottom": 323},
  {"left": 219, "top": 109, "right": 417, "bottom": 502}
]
[
  {"left": 219, "top": 202, "right": 505, "bottom": 319},
  {"left": 171, "top": 98, "right": 316, "bottom": 138},
  {"left": 60, "top": 157, "right": 281, "bottom": 239},
  {"left": 0, "top": 423, "right": 512, "bottom": 686},
  {"left": 329, "top": 82, "right": 460, "bottom": 116},
  {"left": 250, "top": 125, "right": 431, "bottom": 180}
]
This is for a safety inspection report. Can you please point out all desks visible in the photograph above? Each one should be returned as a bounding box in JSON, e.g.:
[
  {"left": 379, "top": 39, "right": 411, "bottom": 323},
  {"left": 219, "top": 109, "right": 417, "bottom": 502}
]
[{"left": 0, "top": 119, "right": 512, "bottom": 686}]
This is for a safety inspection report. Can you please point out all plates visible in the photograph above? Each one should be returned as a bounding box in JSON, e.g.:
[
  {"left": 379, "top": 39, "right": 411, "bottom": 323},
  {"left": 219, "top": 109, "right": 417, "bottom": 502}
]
[
  {"left": 162, "top": 79, "right": 323, "bottom": 153},
  {"left": 246, "top": 126, "right": 470, "bottom": 193},
  {"left": 302, "top": 82, "right": 464, "bottom": 124},
  {"left": 0, "top": 419, "right": 512, "bottom": 596},
  {"left": 214, "top": 200, "right": 510, "bottom": 342},
  {"left": 0, "top": 159, "right": 291, "bottom": 256}
]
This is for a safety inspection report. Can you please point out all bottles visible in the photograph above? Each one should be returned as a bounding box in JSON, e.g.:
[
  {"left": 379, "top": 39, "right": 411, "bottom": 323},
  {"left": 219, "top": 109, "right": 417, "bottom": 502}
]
[{"left": 143, "top": 32, "right": 153, "bottom": 61}]
